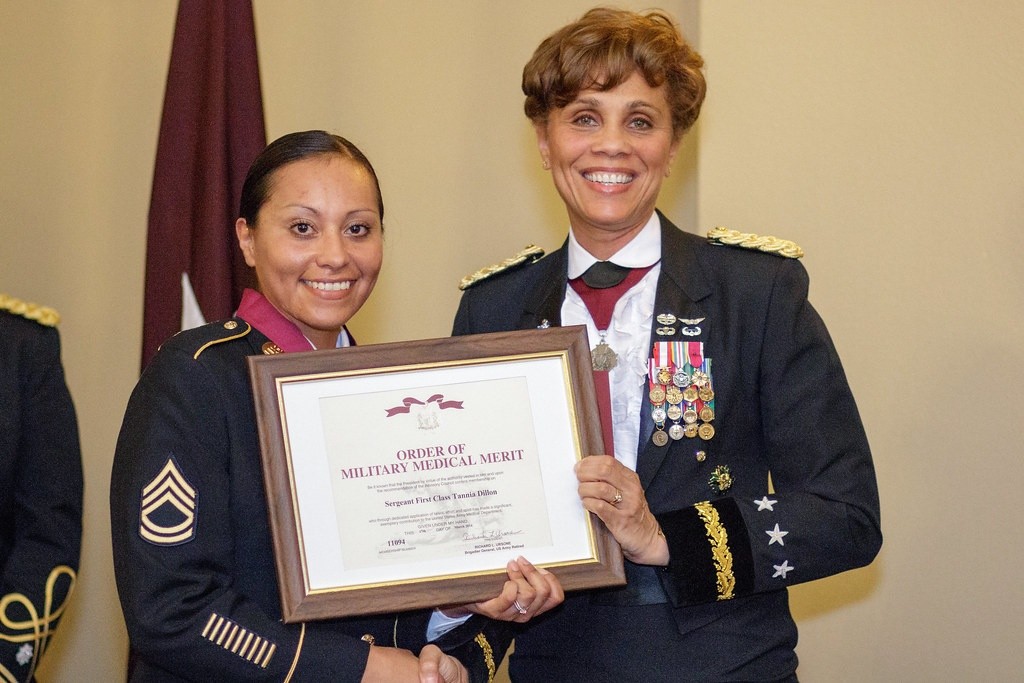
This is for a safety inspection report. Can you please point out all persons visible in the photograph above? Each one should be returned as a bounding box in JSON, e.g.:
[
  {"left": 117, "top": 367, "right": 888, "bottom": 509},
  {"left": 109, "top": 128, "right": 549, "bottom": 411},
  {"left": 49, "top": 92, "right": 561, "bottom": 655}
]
[
  {"left": 110, "top": 131, "right": 564, "bottom": 683},
  {"left": 1, "top": 299, "right": 90, "bottom": 683},
  {"left": 419, "top": 5, "right": 885, "bottom": 683}
]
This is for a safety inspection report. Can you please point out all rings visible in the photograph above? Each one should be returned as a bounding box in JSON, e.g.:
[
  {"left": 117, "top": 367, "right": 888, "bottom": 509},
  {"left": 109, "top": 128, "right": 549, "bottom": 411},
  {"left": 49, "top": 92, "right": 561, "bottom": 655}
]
[
  {"left": 610, "top": 490, "right": 623, "bottom": 505},
  {"left": 514, "top": 600, "right": 527, "bottom": 614}
]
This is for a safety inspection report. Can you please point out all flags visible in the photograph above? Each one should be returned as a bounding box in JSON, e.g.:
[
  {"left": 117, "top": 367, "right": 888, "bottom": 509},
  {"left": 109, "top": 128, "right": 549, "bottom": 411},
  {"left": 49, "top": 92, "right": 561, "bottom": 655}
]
[{"left": 139, "top": 0, "right": 268, "bottom": 378}]
